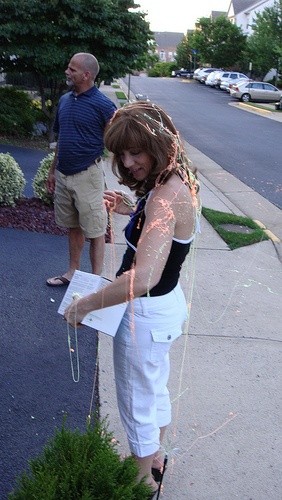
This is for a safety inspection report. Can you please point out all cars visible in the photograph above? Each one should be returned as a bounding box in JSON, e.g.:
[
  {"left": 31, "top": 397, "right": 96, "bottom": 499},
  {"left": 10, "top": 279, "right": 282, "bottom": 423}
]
[
  {"left": 171, "top": 70, "right": 194, "bottom": 79},
  {"left": 229, "top": 81, "right": 282, "bottom": 103}
]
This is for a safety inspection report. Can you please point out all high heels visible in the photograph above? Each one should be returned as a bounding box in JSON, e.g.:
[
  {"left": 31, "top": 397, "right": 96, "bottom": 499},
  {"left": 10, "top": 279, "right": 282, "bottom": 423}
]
[{"left": 146, "top": 455, "right": 166, "bottom": 500}]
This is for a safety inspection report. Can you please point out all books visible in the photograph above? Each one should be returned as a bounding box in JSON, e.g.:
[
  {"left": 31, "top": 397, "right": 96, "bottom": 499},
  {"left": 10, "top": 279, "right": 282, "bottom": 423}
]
[{"left": 57, "top": 269, "right": 129, "bottom": 336}]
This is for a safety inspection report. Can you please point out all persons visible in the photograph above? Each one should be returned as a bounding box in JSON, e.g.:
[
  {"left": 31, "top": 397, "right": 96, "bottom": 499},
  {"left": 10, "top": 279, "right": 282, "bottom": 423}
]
[
  {"left": 64, "top": 101, "right": 200, "bottom": 500},
  {"left": 46, "top": 53, "right": 118, "bottom": 286}
]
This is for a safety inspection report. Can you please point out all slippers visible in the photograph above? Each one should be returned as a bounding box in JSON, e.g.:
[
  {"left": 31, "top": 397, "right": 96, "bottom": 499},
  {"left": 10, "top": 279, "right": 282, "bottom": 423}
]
[{"left": 46, "top": 276, "right": 70, "bottom": 287}]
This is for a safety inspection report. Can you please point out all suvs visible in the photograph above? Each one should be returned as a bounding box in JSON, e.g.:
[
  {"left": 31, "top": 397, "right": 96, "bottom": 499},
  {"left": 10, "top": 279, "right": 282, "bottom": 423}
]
[{"left": 193, "top": 67, "right": 253, "bottom": 93}]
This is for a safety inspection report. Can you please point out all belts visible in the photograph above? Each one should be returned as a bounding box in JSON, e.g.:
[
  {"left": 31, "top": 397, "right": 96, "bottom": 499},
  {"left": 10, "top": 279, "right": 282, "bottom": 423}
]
[{"left": 83, "top": 157, "right": 102, "bottom": 171}]
